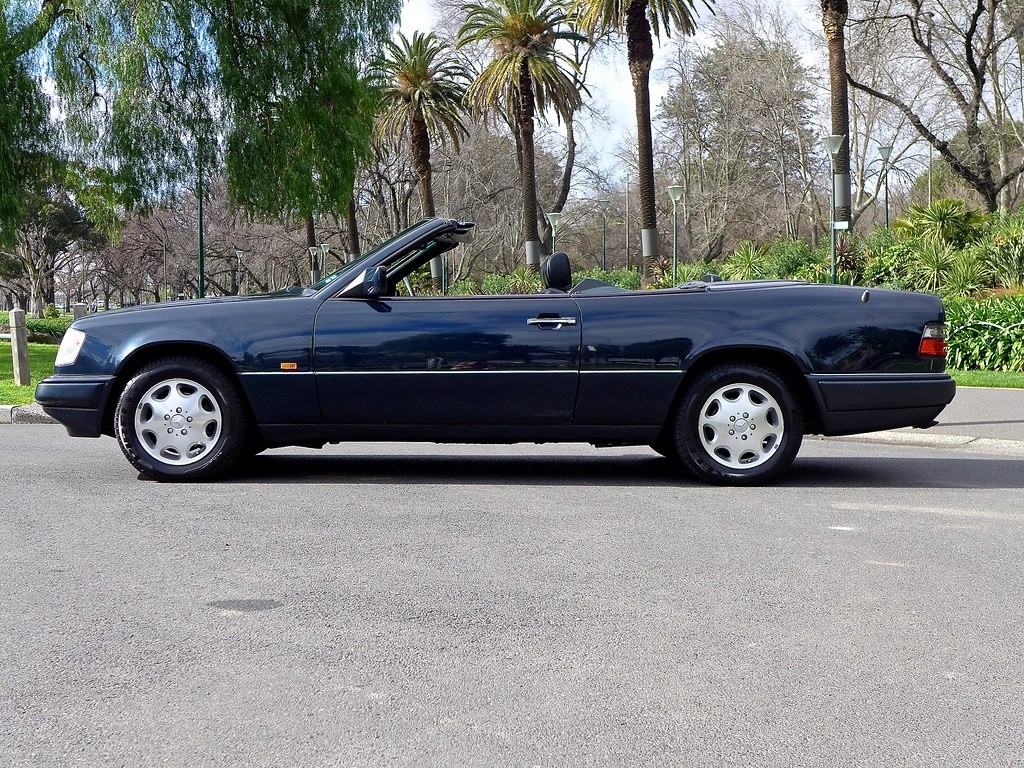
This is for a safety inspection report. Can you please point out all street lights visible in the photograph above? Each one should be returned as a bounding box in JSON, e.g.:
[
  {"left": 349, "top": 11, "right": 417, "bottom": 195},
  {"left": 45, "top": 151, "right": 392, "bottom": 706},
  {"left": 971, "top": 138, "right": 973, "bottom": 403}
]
[
  {"left": 235, "top": 251, "right": 244, "bottom": 296},
  {"left": 666, "top": 185, "right": 684, "bottom": 288},
  {"left": 308, "top": 247, "right": 317, "bottom": 285},
  {"left": 545, "top": 213, "right": 562, "bottom": 253},
  {"left": 320, "top": 243, "right": 330, "bottom": 278},
  {"left": 598, "top": 200, "right": 609, "bottom": 270},
  {"left": 821, "top": 134, "right": 847, "bottom": 284},
  {"left": 878, "top": 146, "right": 893, "bottom": 228}
]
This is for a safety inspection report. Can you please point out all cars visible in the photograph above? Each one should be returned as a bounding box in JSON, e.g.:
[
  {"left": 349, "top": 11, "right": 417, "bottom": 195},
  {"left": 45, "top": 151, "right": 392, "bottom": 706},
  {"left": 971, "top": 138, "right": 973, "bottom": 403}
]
[{"left": 34, "top": 217, "right": 957, "bottom": 488}]
